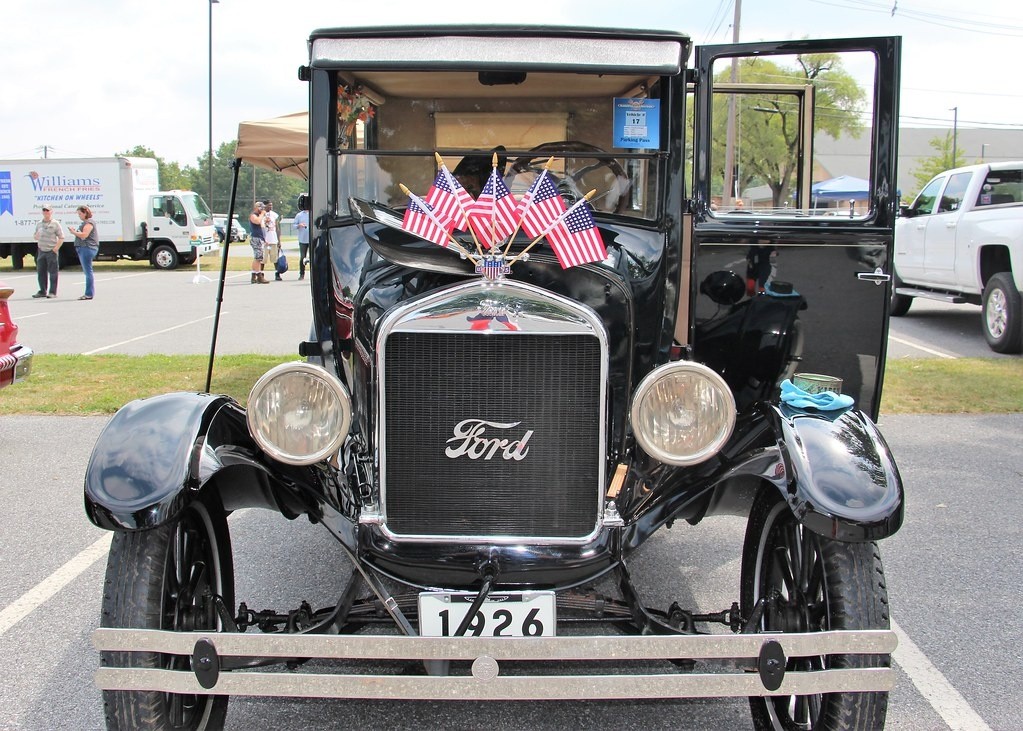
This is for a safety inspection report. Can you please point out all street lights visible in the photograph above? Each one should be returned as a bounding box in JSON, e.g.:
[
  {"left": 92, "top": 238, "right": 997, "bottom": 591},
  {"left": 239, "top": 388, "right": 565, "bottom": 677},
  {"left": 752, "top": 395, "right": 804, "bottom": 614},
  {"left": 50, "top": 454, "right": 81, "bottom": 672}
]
[
  {"left": 208, "top": 0, "right": 220, "bottom": 209},
  {"left": 948, "top": 107, "right": 957, "bottom": 169},
  {"left": 982, "top": 144, "right": 989, "bottom": 159}
]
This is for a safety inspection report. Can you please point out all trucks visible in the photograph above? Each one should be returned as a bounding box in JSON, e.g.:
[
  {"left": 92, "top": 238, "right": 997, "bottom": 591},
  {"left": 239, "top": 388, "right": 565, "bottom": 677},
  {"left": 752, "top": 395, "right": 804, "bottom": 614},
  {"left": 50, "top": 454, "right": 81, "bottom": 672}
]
[
  {"left": 213, "top": 213, "right": 247, "bottom": 243},
  {"left": 0, "top": 156, "right": 221, "bottom": 270}
]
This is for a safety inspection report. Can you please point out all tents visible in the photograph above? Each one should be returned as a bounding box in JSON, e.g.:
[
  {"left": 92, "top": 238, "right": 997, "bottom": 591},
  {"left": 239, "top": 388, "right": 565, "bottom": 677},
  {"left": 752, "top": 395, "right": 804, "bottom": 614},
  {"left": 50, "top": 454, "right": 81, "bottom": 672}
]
[
  {"left": 206, "top": 112, "right": 365, "bottom": 393},
  {"left": 793, "top": 175, "right": 901, "bottom": 215}
]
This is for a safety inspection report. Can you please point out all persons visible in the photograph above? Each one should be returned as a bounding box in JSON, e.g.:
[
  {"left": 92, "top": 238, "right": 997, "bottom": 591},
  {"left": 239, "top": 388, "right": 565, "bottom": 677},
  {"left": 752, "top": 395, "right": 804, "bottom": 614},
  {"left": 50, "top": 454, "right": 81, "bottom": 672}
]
[
  {"left": 31, "top": 206, "right": 64, "bottom": 298},
  {"left": 69, "top": 206, "right": 99, "bottom": 300},
  {"left": 262, "top": 200, "right": 283, "bottom": 281},
  {"left": 735, "top": 200, "right": 743, "bottom": 210},
  {"left": 248, "top": 202, "right": 270, "bottom": 284},
  {"left": 739, "top": 222, "right": 779, "bottom": 298},
  {"left": 292, "top": 210, "right": 309, "bottom": 280}
]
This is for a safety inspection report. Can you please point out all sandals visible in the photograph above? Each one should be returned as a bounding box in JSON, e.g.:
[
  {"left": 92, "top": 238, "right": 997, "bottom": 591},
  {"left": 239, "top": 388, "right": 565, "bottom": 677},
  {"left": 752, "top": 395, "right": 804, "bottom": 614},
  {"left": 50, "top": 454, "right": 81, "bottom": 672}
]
[{"left": 78, "top": 295, "right": 93, "bottom": 300}]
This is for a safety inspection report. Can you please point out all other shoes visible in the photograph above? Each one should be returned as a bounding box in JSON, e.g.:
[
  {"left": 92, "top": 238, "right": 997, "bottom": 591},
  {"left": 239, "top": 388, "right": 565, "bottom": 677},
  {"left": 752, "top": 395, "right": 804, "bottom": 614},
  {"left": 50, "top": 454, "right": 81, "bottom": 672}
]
[
  {"left": 32, "top": 293, "right": 47, "bottom": 298},
  {"left": 298, "top": 274, "right": 304, "bottom": 280},
  {"left": 47, "top": 292, "right": 57, "bottom": 298}
]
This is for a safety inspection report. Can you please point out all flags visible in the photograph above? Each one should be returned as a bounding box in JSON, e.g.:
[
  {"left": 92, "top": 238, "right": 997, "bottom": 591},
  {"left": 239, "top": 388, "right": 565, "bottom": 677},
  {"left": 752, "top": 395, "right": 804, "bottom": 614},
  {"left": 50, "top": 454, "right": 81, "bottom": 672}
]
[
  {"left": 468, "top": 168, "right": 518, "bottom": 249},
  {"left": 403, "top": 194, "right": 456, "bottom": 246},
  {"left": 545, "top": 200, "right": 608, "bottom": 269},
  {"left": 425, "top": 167, "right": 476, "bottom": 232},
  {"left": 514, "top": 171, "right": 566, "bottom": 239}
]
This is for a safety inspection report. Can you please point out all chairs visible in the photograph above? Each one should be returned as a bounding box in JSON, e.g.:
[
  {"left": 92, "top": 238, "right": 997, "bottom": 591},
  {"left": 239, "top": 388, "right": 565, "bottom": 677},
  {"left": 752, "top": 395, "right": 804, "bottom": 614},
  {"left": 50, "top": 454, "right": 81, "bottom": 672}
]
[
  {"left": 990, "top": 193, "right": 1014, "bottom": 205},
  {"left": 167, "top": 199, "right": 182, "bottom": 223}
]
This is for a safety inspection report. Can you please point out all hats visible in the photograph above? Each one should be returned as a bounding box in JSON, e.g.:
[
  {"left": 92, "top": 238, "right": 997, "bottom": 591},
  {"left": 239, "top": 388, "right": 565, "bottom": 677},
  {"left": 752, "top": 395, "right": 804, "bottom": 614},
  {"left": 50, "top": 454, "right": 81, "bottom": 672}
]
[
  {"left": 254, "top": 201, "right": 266, "bottom": 208},
  {"left": 263, "top": 199, "right": 272, "bottom": 205},
  {"left": 42, "top": 203, "right": 52, "bottom": 210}
]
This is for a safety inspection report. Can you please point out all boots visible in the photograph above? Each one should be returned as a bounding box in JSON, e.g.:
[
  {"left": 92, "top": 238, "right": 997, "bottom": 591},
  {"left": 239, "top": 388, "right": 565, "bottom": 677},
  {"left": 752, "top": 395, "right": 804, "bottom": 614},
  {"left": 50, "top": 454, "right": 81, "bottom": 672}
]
[
  {"left": 257, "top": 272, "right": 269, "bottom": 284},
  {"left": 251, "top": 273, "right": 258, "bottom": 283},
  {"left": 275, "top": 272, "right": 282, "bottom": 280}
]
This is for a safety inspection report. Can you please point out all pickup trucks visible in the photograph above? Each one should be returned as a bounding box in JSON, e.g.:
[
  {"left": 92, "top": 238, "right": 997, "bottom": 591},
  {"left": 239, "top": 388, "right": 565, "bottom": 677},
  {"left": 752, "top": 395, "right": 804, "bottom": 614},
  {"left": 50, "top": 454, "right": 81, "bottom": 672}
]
[{"left": 887, "top": 161, "right": 1023, "bottom": 353}]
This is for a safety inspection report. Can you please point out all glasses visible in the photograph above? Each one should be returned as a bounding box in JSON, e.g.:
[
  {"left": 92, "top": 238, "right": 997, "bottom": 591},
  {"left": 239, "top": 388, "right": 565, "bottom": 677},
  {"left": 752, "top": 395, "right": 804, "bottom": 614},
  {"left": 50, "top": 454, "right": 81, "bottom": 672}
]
[{"left": 43, "top": 209, "right": 49, "bottom": 211}]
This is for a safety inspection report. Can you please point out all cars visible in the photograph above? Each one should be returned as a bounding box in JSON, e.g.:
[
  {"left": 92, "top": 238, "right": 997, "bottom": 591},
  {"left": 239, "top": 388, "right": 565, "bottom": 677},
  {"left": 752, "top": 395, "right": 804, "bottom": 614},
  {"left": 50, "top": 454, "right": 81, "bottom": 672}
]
[
  {"left": 83, "top": 26, "right": 905, "bottom": 731},
  {"left": 0, "top": 285, "right": 35, "bottom": 388}
]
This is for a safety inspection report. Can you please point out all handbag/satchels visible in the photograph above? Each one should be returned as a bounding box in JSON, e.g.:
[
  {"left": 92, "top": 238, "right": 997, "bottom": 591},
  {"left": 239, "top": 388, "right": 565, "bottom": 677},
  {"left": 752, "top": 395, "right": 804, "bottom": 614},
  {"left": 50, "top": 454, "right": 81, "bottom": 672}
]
[{"left": 277, "top": 249, "right": 288, "bottom": 273}]
